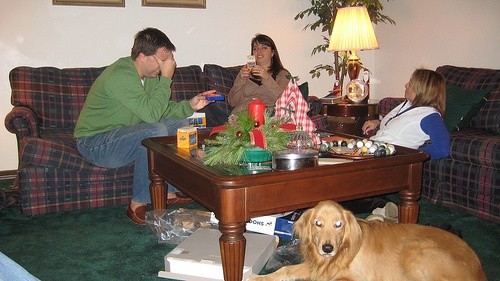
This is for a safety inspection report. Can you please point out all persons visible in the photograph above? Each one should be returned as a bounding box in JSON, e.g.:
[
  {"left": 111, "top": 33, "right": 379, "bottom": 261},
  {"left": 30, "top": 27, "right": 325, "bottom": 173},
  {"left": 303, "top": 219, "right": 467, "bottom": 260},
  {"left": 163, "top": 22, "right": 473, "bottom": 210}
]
[
  {"left": 74, "top": 27, "right": 216, "bottom": 225},
  {"left": 357, "top": 68, "right": 451, "bottom": 212},
  {"left": 206, "top": 34, "right": 292, "bottom": 127}
]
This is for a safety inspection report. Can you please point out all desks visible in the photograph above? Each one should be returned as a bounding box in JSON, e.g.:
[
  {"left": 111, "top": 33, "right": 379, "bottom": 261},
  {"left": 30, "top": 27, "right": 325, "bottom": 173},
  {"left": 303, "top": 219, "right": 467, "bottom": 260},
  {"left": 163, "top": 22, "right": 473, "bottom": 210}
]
[{"left": 141, "top": 128, "right": 429, "bottom": 281}]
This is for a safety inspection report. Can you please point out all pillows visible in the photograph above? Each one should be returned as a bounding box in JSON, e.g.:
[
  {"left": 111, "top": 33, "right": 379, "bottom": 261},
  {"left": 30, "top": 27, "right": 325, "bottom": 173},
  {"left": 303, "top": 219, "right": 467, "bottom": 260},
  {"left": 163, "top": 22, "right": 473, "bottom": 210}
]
[{"left": 444, "top": 84, "right": 491, "bottom": 131}]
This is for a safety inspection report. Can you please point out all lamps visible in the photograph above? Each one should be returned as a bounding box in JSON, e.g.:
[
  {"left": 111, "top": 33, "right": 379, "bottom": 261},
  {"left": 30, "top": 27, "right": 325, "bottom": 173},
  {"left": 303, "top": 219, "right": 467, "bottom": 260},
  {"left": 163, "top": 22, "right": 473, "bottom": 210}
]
[{"left": 328, "top": 6, "right": 379, "bottom": 101}]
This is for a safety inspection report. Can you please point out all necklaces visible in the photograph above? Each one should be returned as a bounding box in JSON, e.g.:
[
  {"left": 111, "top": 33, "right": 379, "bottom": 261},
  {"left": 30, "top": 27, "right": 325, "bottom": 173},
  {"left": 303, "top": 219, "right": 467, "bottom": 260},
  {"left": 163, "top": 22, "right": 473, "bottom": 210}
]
[{"left": 384, "top": 100, "right": 416, "bottom": 127}]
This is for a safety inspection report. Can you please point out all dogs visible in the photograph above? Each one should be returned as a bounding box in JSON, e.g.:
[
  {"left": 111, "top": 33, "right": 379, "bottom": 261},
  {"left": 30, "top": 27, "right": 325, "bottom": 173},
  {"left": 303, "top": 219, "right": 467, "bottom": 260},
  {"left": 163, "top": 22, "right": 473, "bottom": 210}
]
[{"left": 240, "top": 198, "right": 491, "bottom": 281}]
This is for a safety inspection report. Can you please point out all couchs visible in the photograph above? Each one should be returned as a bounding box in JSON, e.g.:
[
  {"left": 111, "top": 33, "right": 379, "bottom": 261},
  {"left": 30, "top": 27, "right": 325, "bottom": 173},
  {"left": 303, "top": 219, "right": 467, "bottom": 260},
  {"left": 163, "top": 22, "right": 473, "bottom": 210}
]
[
  {"left": 5, "top": 64, "right": 328, "bottom": 217},
  {"left": 368, "top": 64, "right": 500, "bottom": 224}
]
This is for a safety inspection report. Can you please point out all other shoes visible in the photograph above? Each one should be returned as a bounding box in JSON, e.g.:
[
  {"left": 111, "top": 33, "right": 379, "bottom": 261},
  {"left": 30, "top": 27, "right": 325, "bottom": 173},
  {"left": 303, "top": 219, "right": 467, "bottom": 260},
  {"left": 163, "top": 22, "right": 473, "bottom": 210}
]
[{"left": 424, "top": 223, "right": 463, "bottom": 238}]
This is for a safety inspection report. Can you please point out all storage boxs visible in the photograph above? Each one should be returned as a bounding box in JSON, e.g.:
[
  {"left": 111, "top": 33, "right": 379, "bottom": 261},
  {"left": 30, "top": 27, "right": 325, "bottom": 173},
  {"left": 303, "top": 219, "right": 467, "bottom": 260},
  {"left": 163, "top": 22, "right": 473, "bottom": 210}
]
[
  {"left": 159, "top": 228, "right": 279, "bottom": 281},
  {"left": 210, "top": 210, "right": 296, "bottom": 240},
  {"left": 187, "top": 112, "right": 207, "bottom": 129},
  {"left": 176, "top": 127, "right": 198, "bottom": 149}
]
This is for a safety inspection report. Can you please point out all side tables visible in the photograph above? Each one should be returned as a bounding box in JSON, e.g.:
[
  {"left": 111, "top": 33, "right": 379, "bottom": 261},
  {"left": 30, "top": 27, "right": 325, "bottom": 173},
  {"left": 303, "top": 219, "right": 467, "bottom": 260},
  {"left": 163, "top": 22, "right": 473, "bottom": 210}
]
[{"left": 320, "top": 98, "right": 379, "bottom": 136}]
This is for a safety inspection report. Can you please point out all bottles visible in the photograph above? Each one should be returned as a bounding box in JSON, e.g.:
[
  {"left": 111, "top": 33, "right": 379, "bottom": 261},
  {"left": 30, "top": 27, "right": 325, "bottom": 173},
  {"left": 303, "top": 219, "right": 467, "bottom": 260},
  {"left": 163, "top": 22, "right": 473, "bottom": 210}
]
[{"left": 248, "top": 99, "right": 265, "bottom": 127}]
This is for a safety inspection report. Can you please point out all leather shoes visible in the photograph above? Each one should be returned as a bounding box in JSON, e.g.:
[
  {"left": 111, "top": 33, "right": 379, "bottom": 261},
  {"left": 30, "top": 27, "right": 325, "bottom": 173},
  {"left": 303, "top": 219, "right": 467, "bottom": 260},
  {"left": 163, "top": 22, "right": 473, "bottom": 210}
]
[
  {"left": 167, "top": 192, "right": 193, "bottom": 205},
  {"left": 126, "top": 205, "right": 151, "bottom": 225}
]
